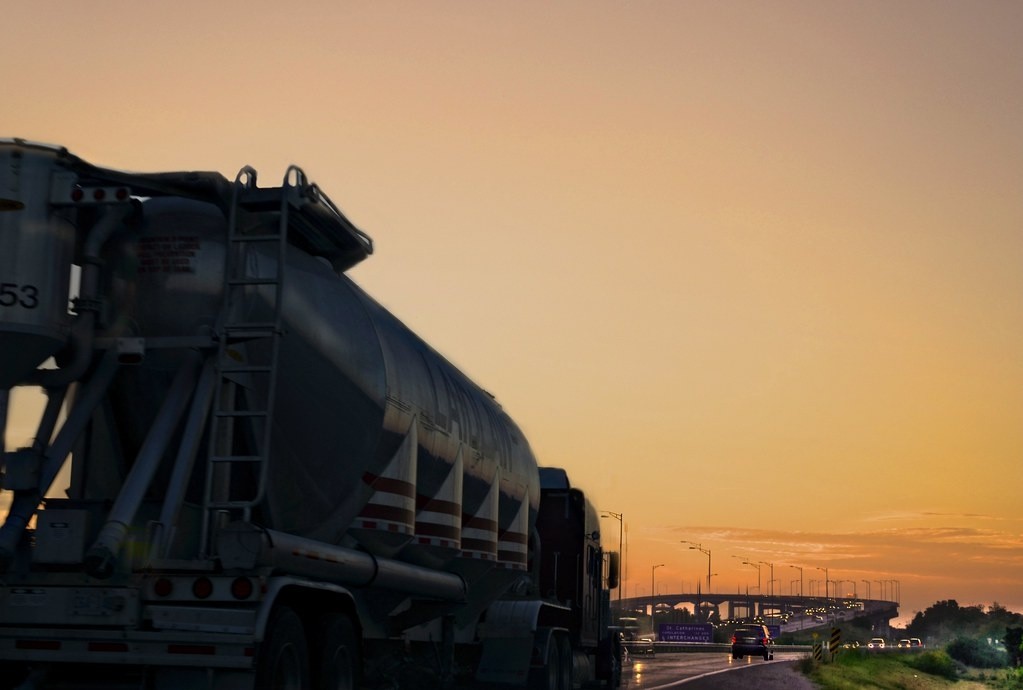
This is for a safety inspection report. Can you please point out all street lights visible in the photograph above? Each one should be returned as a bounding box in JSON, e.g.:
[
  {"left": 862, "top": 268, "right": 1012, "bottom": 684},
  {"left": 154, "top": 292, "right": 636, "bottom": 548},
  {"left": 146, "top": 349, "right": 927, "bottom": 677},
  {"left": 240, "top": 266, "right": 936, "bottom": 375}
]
[
  {"left": 742, "top": 561, "right": 761, "bottom": 594},
  {"left": 681, "top": 540, "right": 711, "bottom": 592},
  {"left": 759, "top": 561, "right": 773, "bottom": 625},
  {"left": 600, "top": 513, "right": 623, "bottom": 623},
  {"left": 706, "top": 573, "right": 718, "bottom": 592},
  {"left": 816, "top": 567, "right": 828, "bottom": 621},
  {"left": 790, "top": 565, "right": 803, "bottom": 629},
  {"left": 651, "top": 564, "right": 664, "bottom": 630}
]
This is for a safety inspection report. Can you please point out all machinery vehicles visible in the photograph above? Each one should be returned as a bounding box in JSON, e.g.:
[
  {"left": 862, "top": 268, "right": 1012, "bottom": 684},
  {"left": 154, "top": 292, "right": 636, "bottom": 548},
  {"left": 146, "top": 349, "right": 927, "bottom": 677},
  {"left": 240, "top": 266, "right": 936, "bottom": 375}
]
[{"left": 0, "top": 137, "right": 623, "bottom": 690}]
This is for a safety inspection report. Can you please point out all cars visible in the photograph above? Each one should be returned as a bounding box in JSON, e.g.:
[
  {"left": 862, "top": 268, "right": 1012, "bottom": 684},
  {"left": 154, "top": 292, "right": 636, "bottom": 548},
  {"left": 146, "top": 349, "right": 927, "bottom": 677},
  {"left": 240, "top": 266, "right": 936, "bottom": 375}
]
[
  {"left": 830, "top": 579, "right": 901, "bottom": 613},
  {"left": 897, "top": 639, "right": 911, "bottom": 648},
  {"left": 746, "top": 579, "right": 823, "bottom": 597},
  {"left": 911, "top": 638, "right": 921, "bottom": 647},
  {"left": 732, "top": 624, "right": 773, "bottom": 661},
  {"left": 868, "top": 638, "right": 885, "bottom": 648}
]
